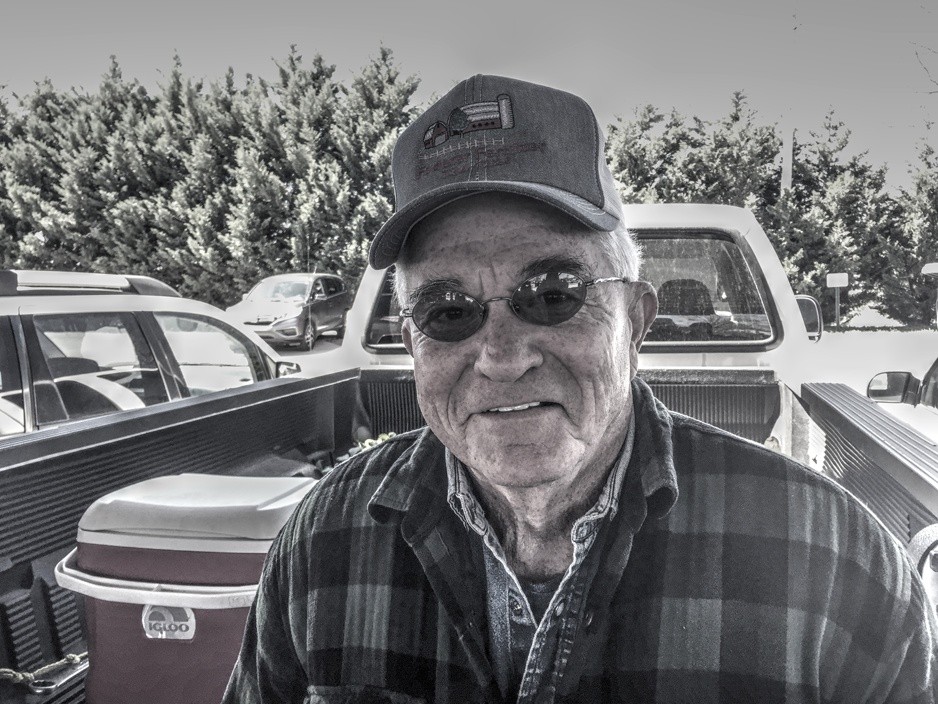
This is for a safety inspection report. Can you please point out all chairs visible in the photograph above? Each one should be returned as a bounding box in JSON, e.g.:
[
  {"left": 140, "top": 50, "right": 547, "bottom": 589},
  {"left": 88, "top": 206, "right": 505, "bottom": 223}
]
[{"left": 656, "top": 279, "right": 718, "bottom": 342}]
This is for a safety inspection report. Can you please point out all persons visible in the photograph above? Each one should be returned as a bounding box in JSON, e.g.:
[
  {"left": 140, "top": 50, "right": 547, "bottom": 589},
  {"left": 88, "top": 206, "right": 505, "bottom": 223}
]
[{"left": 215, "top": 74, "right": 937, "bottom": 704}]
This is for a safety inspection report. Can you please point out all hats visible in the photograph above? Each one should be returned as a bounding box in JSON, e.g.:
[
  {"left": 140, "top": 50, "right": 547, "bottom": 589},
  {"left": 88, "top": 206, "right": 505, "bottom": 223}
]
[{"left": 367, "top": 74, "right": 623, "bottom": 270}]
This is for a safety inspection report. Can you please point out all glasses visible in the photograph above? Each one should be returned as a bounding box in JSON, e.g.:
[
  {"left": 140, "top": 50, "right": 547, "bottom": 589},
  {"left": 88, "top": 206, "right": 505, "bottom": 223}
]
[{"left": 401, "top": 271, "right": 630, "bottom": 342}]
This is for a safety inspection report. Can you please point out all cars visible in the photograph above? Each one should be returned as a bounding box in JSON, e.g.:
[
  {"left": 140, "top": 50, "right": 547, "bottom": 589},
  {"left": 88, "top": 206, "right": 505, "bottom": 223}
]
[{"left": 222, "top": 273, "right": 350, "bottom": 352}]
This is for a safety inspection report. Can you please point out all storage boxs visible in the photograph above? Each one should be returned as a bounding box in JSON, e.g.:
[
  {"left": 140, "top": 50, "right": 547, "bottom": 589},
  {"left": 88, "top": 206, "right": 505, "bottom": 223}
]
[{"left": 53, "top": 471, "right": 317, "bottom": 704}]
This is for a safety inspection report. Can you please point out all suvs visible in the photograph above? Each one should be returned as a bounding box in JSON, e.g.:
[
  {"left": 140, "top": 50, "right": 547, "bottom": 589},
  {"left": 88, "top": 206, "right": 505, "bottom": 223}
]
[
  {"left": 0, "top": 265, "right": 305, "bottom": 438},
  {"left": 348, "top": 203, "right": 825, "bottom": 461}
]
